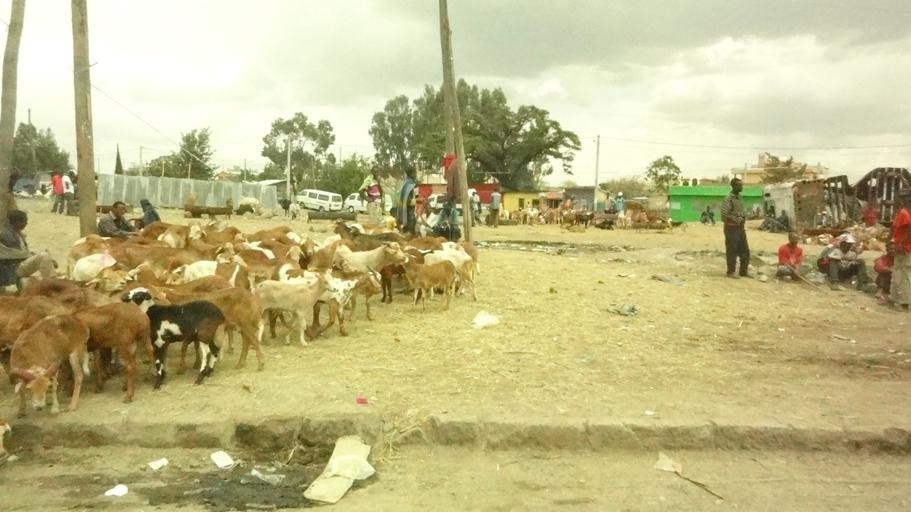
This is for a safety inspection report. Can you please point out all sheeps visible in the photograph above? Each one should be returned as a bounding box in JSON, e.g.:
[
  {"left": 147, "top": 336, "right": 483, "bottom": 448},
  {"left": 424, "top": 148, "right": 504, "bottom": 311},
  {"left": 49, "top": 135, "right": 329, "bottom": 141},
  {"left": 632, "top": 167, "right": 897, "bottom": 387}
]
[
  {"left": 498, "top": 208, "right": 626, "bottom": 231},
  {"left": 1, "top": 215, "right": 480, "bottom": 419},
  {"left": 235, "top": 195, "right": 302, "bottom": 220}
]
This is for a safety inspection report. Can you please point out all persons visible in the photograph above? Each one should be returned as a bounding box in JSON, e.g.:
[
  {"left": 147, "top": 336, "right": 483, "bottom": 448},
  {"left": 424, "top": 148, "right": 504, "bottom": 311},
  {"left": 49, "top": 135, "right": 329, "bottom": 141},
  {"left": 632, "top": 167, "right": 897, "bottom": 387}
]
[
  {"left": 758, "top": 192, "right": 911, "bottom": 312},
  {"left": 0, "top": 210, "right": 58, "bottom": 283},
  {"left": 227, "top": 197, "right": 234, "bottom": 220},
  {"left": 6, "top": 168, "right": 23, "bottom": 220},
  {"left": 604, "top": 192, "right": 626, "bottom": 229},
  {"left": 719, "top": 177, "right": 754, "bottom": 278},
  {"left": 470, "top": 191, "right": 480, "bottom": 221},
  {"left": 282, "top": 198, "right": 301, "bottom": 220},
  {"left": 135, "top": 198, "right": 161, "bottom": 230},
  {"left": 563, "top": 193, "right": 576, "bottom": 209},
  {"left": 358, "top": 167, "right": 462, "bottom": 243},
  {"left": 98, "top": 202, "right": 143, "bottom": 240},
  {"left": 50, "top": 170, "right": 79, "bottom": 215},
  {"left": 489, "top": 187, "right": 502, "bottom": 229},
  {"left": 701, "top": 205, "right": 716, "bottom": 225}
]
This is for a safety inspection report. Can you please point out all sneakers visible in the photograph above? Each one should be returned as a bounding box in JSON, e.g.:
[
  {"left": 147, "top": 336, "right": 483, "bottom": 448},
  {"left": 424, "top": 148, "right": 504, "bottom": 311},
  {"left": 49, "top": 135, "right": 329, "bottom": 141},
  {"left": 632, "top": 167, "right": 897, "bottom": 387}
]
[{"left": 828, "top": 281, "right": 873, "bottom": 293}]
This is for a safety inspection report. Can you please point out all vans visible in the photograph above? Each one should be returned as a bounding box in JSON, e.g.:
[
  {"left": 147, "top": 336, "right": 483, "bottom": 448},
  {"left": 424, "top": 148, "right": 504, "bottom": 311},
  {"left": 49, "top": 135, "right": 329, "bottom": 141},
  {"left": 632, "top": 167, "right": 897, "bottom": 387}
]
[{"left": 294, "top": 185, "right": 490, "bottom": 219}]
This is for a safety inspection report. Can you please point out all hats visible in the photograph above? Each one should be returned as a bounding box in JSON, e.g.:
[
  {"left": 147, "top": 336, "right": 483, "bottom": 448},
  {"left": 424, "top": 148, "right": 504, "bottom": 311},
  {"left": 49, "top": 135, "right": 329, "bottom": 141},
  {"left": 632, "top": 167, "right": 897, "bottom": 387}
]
[
  {"left": 7, "top": 209, "right": 26, "bottom": 222},
  {"left": 788, "top": 231, "right": 801, "bottom": 241},
  {"left": 841, "top": 234, "right": 856, "bottom": 244}
]
